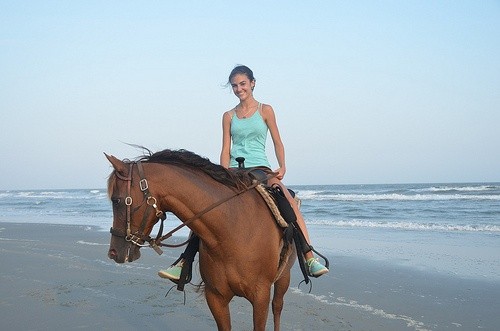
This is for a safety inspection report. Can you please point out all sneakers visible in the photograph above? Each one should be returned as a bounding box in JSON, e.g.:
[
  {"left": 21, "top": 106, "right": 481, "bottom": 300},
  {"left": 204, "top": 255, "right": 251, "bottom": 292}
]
[
  {"left": 305, "top": 258, "right": 329, "bottom": 275},
  {"left": 158, "top": 265, "right": 183, "bottom": 280}
]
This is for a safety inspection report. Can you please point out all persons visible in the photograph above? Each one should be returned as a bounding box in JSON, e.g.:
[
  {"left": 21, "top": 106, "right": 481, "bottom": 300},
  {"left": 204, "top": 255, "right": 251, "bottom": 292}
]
[{"left": 157, "top": 65, "right": 329, "bottom": 281}]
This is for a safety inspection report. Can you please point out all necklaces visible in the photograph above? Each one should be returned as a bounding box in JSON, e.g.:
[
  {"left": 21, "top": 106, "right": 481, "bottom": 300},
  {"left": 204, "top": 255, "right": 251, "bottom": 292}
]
[{"left": 237, "top": 105, "right": 254, "bottom": 119}]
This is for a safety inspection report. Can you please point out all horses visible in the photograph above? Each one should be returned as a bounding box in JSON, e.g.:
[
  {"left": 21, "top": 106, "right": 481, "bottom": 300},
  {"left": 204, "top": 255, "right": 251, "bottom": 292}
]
[{"left": 103, "top": 140, "right": 302, "bottom": 331}]
